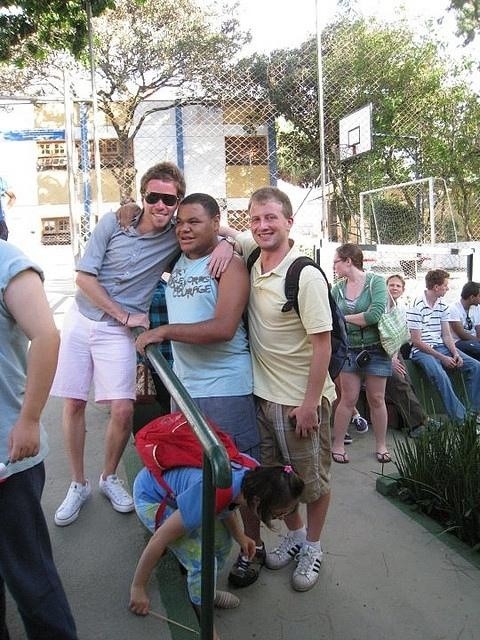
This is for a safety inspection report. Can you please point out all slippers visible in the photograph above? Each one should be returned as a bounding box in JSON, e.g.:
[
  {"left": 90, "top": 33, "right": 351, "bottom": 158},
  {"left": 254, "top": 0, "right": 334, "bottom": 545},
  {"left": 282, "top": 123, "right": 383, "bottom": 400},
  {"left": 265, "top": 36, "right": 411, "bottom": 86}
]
[
  {"left": 375, "top": 450, "right": 392, "bottom": 463},
  {"left": 332, "top": 451, "right": 350, "bottom": 464}
]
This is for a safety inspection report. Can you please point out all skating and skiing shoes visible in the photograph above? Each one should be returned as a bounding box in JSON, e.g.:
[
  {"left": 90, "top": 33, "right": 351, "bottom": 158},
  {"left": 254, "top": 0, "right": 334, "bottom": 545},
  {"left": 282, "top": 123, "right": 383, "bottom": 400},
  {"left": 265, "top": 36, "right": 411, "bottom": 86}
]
[{"left": 53, "top": 478, "right": 92, "bottom": 527}]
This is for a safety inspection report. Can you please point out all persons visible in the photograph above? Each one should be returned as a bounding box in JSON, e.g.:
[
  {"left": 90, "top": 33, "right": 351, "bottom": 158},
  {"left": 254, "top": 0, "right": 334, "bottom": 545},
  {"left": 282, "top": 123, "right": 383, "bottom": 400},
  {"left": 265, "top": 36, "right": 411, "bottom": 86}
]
[
  {"left": 383, "top": 269, "right": 480, "bottom": 440},
  {"left": 128, "top": 452, "right": 304, "bottom": 615},
  {"left": 115, "top": 193, "right": 266, "bottom": 590},
  {"left": 0, "top": 221, "right": 78, "bottom": 639},
  {"left": 49, "top": 162, "right": 242, "bottom": 526},
  {"left": 330, "top": 243, "right": 392, "bottom": 464},
  {"left": 219, "top": 186, "right": 339, "bottom": 590}
]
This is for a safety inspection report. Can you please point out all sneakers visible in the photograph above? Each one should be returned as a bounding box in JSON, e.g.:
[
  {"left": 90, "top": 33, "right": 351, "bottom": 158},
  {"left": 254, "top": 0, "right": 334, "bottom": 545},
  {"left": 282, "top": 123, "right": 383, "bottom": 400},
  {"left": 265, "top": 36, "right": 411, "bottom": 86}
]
[
  {"left": 214, "top": 590, "right": 241, "bottom": 610},
  {"left": 343, "top": 432, "right": 353, "bottom": 444},
  {"left": 454, "top": 422, "right": 480, "bottom": 435},
  {"left": 97, "top": 470, "right": 135, "bottom": 514},
  {"left": 265, "top": 529, "right": 308, "bottom": 571},
  {"left": 291, "top": 543, "right": 323, "bottom": 593},
  {"left": 352, "top": 413, "right": 369, "bottom": 434},
  {"left": 408, "top": 417, "right": 446, "bottom": 439},
  {"left": 227, "top": 540, "right": 267, "bottom": 588},
  {"left": 475, "top": 415, "right": 480, "bottom": 424}
]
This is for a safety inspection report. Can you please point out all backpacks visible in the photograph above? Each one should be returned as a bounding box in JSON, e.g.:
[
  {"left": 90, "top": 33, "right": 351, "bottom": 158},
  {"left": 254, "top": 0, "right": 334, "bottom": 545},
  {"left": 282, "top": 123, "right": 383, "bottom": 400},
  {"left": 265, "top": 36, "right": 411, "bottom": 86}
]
[
  {"left": 241, "top": 243, "right": 350, "bottom": 384},
  {"left": 132, "top": 408, "right": 261, "bottom": 518}
]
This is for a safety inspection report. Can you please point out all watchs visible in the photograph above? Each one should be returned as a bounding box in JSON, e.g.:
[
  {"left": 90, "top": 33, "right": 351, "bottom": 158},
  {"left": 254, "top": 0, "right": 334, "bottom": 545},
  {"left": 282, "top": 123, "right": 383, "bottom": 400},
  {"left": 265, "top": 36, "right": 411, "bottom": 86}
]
[{"left": 222, "top": 236, "right": 236, "bottom": 246}]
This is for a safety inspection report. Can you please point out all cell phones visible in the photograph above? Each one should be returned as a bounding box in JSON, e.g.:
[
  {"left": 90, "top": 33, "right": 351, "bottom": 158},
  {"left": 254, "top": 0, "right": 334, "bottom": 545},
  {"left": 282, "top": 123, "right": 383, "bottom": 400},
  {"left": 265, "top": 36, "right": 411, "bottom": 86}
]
[{"left": 288, "top": 415, "right": 321, "bottom": 427}]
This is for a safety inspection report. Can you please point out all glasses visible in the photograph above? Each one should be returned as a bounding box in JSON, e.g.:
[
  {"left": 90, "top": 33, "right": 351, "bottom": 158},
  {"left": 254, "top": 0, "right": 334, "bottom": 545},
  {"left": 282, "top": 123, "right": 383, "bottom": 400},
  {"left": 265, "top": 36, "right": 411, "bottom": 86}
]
[
  {"left": 141, "top": 190, "right": 179, "bottom": 207},
  {"left": 465, "top": 316, "right": 474, "bottom": 330}
]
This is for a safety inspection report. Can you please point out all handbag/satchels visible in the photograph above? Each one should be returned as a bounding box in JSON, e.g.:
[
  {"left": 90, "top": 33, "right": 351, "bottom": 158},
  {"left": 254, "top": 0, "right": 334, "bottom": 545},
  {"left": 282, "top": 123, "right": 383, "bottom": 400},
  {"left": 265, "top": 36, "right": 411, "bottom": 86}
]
[{"left": 368, "top": 274, "right": 413, "bottom": 358}]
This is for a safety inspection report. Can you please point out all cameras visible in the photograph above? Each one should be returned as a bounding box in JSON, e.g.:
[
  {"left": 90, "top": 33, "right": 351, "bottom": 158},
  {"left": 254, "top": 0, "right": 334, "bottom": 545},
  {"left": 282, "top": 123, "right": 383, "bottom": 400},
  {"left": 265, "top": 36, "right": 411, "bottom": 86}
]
[{"left": 353, "top": 350, "right": 371, "bottom": 368}]
